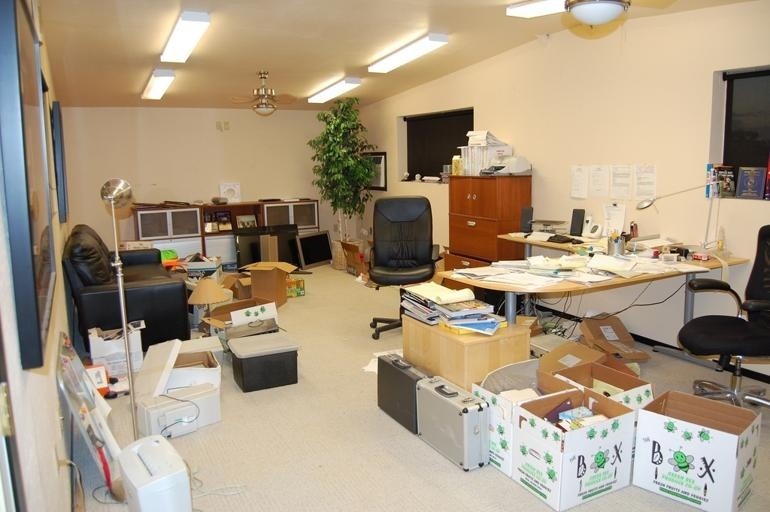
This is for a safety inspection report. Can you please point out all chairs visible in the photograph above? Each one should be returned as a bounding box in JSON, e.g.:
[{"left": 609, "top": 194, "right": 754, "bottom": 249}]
[
  {"left": 675, "top": 223, "right": 770, "bottom": 408},
  {"left": 368, "top": 196, "right": 440, "bottom": 340}
]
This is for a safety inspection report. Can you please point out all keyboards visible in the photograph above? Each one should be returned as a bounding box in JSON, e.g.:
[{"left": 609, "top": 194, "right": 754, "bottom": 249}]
[{"left": 524, "top": 233, "right": 574, "bottom": 242}]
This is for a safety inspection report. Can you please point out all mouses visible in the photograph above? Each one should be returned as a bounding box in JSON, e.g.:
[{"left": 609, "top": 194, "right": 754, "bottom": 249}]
[{"left": 572, "top": 240, "right": 584, "bottom": 244}]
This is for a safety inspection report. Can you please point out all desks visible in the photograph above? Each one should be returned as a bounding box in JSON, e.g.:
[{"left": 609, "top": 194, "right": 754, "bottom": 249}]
[{"left": 434, "top": 228, "right": 745, "bottom": 371}]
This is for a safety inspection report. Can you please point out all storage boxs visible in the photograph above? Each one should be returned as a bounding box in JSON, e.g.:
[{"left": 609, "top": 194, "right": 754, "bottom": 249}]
[
  {"left": 89, "top": 319, "right": 224, "bottom": 512},
  {"left": 184, "top": 254, "right": 305, "bottom": 391},
  {"left": 343, "top": 237, "right": 373, "bottom": 279}
]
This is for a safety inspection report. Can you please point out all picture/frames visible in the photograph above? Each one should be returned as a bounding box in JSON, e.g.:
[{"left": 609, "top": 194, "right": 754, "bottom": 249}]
[{"left": 362, "top": 151, "right": 387, "bottom": 190}]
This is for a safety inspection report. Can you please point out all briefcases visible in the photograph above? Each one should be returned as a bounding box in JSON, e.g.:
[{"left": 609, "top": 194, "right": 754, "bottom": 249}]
[
  {"left": 415, "top": 376, "right": 490, "bottom": 472},
  {"left": 378, "top": 354, "right": 433, "bottom": 435}
]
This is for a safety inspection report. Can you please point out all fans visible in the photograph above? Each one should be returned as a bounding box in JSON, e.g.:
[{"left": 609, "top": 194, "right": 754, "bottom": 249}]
[{"left": 230, "top": 71, "right": 299, "bottom": 107}]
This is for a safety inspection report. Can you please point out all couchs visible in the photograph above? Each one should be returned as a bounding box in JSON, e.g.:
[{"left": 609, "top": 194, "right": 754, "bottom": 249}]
[{"left": 61, "top": 224, "right": 190, "bottom": 353}]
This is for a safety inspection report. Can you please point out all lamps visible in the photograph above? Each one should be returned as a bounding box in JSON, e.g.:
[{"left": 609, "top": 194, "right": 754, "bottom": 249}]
[
  {"left": 367, "top": 33, "right": 447, "bottom": 74},
  {"left": 141, "top": 70, "right": 175, "bottom": 100},
  {"left": 505, "top": 0, "right": 565, "bottom": 18},
  {"left": 565, "top": 0, "right": 632, "bottom": 25},
  {"left": 100, "top": 177, "right": 141, "bottom": 443},
  {"left": 309, "top": 77, "right": 361, "bottom": 102},
  {"left": 161, "top": 12, "right": 210, "bottom": 63},
  {"left": 633, "top": 178, "right": 714, "bottom": 254},
  {"left": 250, "top": 70, "right": 276, "bottom": 114}
]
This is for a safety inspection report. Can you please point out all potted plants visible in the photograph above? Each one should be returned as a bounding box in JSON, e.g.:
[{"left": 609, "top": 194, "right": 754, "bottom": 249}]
[{"left": 305, "top": 95, "right": 380, "bottom": 273}]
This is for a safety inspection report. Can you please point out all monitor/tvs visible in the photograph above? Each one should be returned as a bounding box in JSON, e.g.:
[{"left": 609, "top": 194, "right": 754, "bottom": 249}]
[{"left": 294, "top": 229, "right": 334, "bottom": 270}]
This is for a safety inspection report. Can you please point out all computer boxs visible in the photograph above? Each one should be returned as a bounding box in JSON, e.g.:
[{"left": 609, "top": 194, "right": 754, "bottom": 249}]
[{"left": 528, "top": 330, "right": 568, "bottom": 357}]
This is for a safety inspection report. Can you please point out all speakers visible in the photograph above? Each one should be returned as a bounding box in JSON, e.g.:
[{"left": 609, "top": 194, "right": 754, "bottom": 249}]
[
  {"left": 570, "top": 209, "right": 585, "bottom": 237},
  {"left": 520, "top": 208, "right": 533, "bottom": 233}
]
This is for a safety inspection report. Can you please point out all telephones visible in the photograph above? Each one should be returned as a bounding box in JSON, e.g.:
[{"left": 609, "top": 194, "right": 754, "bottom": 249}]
[{"left": 582, "top": 216, "right": 603, "bottom": 239}]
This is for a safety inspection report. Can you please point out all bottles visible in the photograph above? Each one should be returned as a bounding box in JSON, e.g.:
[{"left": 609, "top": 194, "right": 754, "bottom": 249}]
[
  {"left": 630, "top": 221, "right": 637, "bottom": 237},
  {"left": 717, "top": 226, "right": 726, "bottom": 257}
]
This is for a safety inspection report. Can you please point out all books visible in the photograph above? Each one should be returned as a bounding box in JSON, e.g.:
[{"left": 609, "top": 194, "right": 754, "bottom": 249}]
[
  {"left": 705, "top": 163, "right": 767, "bottom": 200},
  {"left": 457, "top": 130, "right": 513, "bottom": 176},
  {"left": 399, "top": 282, "right": 507, "bottom": 334}
]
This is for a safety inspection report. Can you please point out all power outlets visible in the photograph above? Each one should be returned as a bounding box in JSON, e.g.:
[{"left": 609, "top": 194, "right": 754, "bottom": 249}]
[{"left": 55, "top": 446, "right": 60, "bottom": 476}]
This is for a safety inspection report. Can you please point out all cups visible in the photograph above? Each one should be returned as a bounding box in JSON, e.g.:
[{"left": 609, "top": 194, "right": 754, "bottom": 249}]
[
  {"left": 443, "top": 165, "right": 452, "bottom": 174},
  {"left": 607, "top": 236, "right": 625, "bottom": 256}
]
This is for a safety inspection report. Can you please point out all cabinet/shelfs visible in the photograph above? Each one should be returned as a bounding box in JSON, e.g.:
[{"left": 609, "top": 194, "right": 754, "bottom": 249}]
[
  {"left": 445, "top": 175, "right": 531, "bottom": 292},
  {"left": 401, "top": 314, "right": 532, "bottom": 394},
  {"left": 132, "top": 199, "right": 319, "bottom": 267}
]
